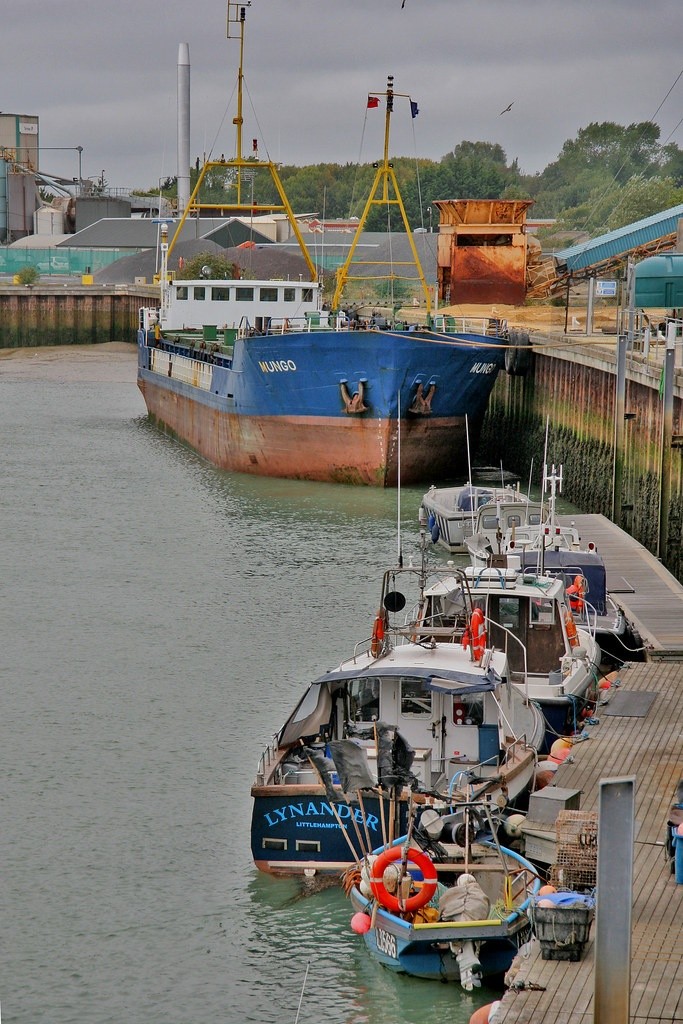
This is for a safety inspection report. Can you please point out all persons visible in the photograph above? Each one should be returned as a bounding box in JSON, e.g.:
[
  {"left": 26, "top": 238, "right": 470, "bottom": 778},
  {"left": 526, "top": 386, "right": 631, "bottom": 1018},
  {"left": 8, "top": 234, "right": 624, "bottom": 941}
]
[{"left": 322, "top": 302, "right": 428, "bottom": 334}]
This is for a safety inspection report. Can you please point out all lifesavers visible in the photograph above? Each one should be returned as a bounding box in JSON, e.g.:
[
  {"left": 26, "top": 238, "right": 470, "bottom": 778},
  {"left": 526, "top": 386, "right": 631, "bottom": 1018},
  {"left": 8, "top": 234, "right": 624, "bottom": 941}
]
[
  {"left": 574, "top": 576, "right": 584, "bottom": 613},
  {"left": 471, "top": 608, "right": 486, "bottom": 660},
  {"left": 564, "top": 610, "right": 576, "bottom": 646},
  {"left": 369, "top": 846, "right": 437, "bottom": 913},
  {"left": 371, "top": 607, "right": 386, "bottom": 658}
]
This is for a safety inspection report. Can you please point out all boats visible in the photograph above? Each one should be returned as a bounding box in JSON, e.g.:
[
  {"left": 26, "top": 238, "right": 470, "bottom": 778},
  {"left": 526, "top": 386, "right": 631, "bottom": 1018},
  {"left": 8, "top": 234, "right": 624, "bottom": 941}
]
[
  {"left": 130, "top": 0, "right": 533, "bottom": 486},
  {"left": 296, "top": 715, "right": 546, "bottom": 991},
  {"left": 231, "top": 387, "right": 651, "bottom": 885}
]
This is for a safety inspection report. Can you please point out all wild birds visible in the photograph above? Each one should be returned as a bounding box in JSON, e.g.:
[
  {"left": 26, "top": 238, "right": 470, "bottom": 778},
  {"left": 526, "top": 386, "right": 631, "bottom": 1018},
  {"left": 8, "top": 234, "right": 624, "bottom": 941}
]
[{"left": 499, "top": 101, "right": 516, "bottom": 116}]
